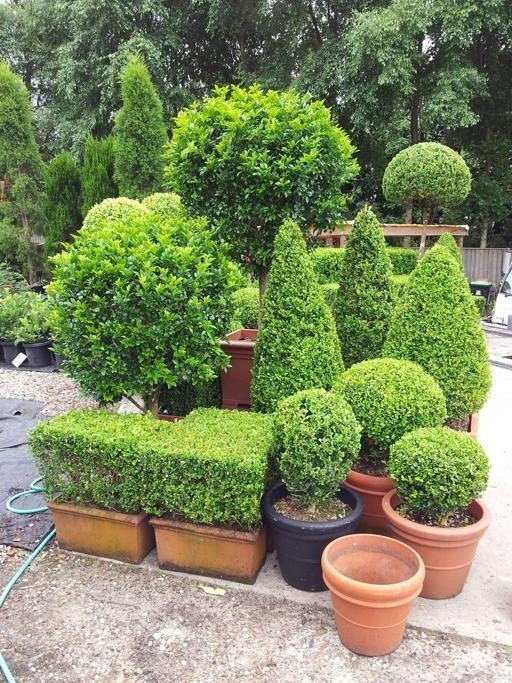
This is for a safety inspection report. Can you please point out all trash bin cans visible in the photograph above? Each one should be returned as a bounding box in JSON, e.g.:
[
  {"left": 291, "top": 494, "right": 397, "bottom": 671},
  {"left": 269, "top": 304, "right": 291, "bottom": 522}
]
[{"left": 469, "top": 281, "right": 493, "bottom": 303}]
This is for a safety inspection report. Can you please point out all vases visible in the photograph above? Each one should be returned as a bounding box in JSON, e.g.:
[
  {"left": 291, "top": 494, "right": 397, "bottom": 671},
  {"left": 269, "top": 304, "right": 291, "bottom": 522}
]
[{"left": 318, "top": 534, "right": 428, "bottom": 655}]
